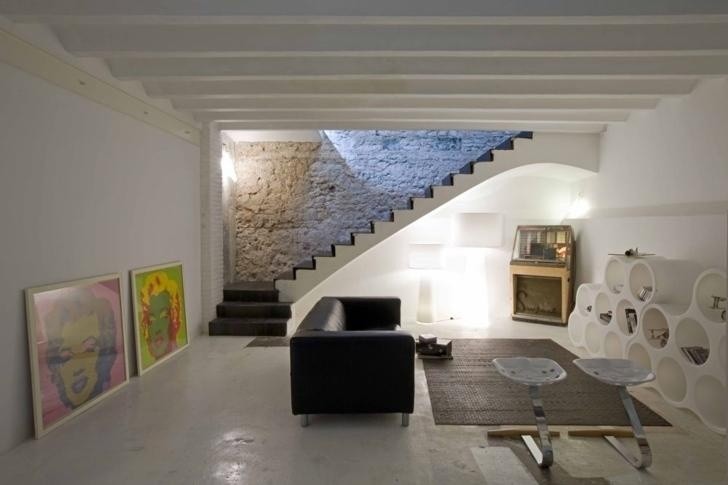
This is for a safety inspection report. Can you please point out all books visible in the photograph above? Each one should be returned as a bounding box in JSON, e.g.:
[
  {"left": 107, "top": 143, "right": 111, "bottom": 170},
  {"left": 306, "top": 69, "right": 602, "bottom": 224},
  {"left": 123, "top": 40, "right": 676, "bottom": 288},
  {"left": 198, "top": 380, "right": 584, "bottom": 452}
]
[
  {"left": 418, "top": 352, "right": 454, "bottom": 360},
  {"left": 599, "top": 286, "right": 709, "bottom": 364}
]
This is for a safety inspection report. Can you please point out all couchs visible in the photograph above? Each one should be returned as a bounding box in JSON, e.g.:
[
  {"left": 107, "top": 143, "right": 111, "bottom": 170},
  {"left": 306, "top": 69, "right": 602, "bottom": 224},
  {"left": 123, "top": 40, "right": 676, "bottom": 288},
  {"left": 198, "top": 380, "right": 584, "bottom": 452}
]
[{"left": 290, "top": 296, "right": 415, "bottom": 427}]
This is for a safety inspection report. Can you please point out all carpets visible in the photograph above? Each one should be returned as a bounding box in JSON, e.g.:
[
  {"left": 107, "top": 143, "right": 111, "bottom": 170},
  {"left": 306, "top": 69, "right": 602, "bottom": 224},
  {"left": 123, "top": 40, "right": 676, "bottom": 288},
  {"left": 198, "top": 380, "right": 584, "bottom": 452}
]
[{"left": 423, "top": 339, "right": 673, "bottom": 426}]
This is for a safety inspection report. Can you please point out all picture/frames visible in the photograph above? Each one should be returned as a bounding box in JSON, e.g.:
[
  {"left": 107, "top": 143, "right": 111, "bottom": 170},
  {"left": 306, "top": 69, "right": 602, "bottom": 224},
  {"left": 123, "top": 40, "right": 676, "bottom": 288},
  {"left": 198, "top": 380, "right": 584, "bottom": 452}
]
[{"left": 23, "top": 260, "right": 191, "bottom": 440}]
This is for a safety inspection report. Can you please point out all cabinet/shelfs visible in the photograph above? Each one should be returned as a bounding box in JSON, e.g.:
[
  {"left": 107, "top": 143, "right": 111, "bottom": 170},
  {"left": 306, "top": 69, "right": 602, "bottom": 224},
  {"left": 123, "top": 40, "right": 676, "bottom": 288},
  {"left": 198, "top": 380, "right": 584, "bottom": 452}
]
[{"left": 509, "top": 225, "right": 576, "bottom": 326}]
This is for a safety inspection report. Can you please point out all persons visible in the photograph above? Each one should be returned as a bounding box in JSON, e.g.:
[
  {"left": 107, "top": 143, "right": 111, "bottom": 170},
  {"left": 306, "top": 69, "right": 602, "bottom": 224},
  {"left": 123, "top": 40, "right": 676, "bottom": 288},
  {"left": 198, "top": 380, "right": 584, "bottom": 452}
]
[
  {"left": 43, "top": 287, "right": 119, "bottom": 412},
  {"left": 140, "top": 272, "right": 181, "bottom": 360}
]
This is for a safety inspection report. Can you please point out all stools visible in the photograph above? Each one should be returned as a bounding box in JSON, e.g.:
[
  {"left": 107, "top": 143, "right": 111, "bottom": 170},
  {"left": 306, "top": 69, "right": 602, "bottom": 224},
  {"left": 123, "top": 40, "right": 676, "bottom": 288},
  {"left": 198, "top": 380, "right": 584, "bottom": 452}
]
[{"left": 487, "top": 356, "right": 656, "bottom": 470}]
